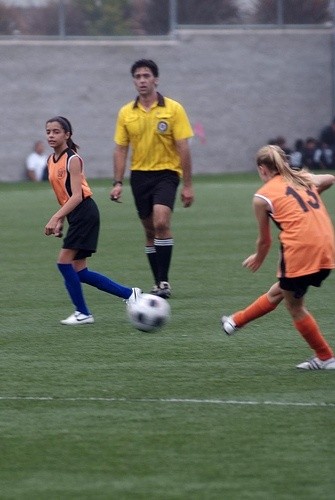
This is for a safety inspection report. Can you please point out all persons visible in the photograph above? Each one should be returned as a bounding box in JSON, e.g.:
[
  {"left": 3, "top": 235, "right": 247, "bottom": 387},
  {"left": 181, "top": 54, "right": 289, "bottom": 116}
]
[
  {"left": 26, "top": 140, "right": 49, "bottom": 183},
  {"left": 44, "top": 116, "right": 141, "bottom": 326},
  {"left": 111, "top": 59, "right": 195, "bottom": 298},
  {"left": 221, "top": 145, "right": 335, "bottom": 370},
  {"left": 269, "top": 120, "right": 335, "bottom": 173}
]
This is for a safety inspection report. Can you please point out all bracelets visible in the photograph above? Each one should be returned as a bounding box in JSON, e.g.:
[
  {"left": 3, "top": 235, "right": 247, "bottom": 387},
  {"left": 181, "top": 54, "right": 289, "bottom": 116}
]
[{"left": 113, "top": 181, "right": 123, "bottom": 186}]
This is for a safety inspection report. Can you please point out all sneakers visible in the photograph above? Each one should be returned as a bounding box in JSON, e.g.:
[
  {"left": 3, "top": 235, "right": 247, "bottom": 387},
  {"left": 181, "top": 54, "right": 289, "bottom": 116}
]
[
  {"left": 296, "top": 356, "right": 335, "bottom": 371},
  {"left": 221, "top": 314, "right": 236, "bottom": 337},
  {"left": 59, "top": 311, "right": 97, "bottom": 326},
  {"left": 151, "top": 281, "right": 171, "bottom": 297},
  {"left": 127, "top": 287, "right": 143, "bottom": 310}
]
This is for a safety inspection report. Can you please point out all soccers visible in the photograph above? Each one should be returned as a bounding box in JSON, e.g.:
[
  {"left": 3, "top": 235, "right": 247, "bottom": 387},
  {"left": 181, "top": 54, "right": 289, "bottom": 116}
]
[{"left": 127, "top": 293, "right": 171, "bottom": 334}]
